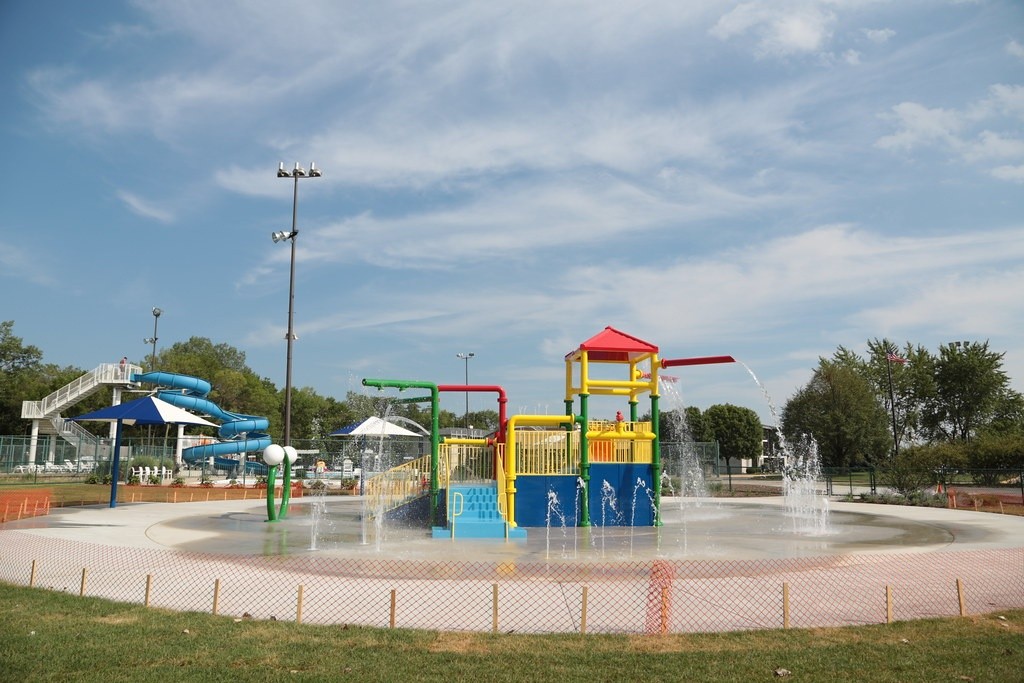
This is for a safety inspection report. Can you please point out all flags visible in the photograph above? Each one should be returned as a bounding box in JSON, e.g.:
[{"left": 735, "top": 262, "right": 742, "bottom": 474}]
[{"left": 886, "top": 348, "right": 910, "bottom": 362}]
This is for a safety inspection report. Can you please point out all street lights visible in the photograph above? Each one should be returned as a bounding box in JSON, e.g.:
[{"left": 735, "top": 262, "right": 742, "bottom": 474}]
[
  {"left": 275, "top": 159, "right": 323, "bottom": 444},
  {"left": 457, "top": 350, "right": 475, "bottom": 428},
  {"left": 143, "top": 308, "right": 162, "bottom": 391}
]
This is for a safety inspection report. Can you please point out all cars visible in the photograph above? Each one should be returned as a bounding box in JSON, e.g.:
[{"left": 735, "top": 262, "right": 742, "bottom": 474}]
[{"left": 13, "top": 457, "right": 135, "bottom": 480}]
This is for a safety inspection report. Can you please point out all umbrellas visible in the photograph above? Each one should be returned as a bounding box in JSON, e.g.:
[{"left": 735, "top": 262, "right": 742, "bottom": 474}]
[
  {"left": 328, "top": 415, "right": 423, "bottom": 518},
  {"left": 64, "top": 395, "right": 221, "bottom": 508}
]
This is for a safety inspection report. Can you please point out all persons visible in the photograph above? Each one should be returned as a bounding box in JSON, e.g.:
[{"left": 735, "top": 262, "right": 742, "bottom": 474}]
[
  {"left": 316, "top": 459, "right": 325, "bottom": 479},
  {"left": 120, "top": 356, "right": 127, "bottom": 380},
  {"left": 277, "top": 464, "right": 280, "bottom": 476}
]
